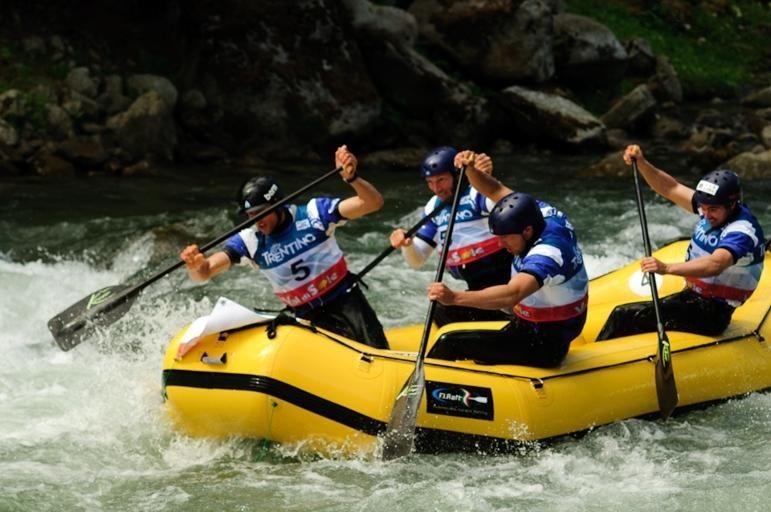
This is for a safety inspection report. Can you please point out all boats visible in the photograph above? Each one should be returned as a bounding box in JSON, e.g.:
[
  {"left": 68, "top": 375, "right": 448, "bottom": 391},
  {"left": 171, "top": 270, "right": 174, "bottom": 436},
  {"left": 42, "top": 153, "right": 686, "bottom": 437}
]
[{"left": 151, "top": 223, "right": 771, "bottom": 457}]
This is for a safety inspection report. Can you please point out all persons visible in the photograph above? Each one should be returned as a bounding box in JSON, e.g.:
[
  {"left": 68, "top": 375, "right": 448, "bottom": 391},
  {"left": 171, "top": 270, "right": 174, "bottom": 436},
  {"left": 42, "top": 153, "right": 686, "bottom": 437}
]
[
  {"left": 389, "top": 141, "right": 514, "bottom": 327},
  {"left": 593, "top": 143, "right": 765, "bottom": 342},
  {"left": 418, "top": 149, "right": 589, "bottom": 369},
  {"left": 181, "top": 144, "right": 392, "bottom": 352}
]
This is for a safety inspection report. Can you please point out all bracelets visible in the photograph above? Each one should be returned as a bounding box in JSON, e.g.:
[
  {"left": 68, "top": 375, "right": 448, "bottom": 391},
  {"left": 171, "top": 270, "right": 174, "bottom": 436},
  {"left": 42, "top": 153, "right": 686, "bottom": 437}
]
[{"left": 343, "top": 172, "right": 358, "bottom": 184}]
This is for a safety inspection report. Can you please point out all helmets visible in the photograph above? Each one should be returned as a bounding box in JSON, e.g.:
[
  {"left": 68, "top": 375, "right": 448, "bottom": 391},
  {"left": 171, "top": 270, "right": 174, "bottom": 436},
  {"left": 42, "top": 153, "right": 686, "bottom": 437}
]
[
  {"left": 692, "top": 169, "right": 743, "bottom": 217},
  {"left": 489, "top": 192, "right": 544, "bottom": 236},
  {"left": 420, "top": 145, "right": 469, "bottom": 197},
  {"left": 237, "top": 175, "right": 286, "bottom": 217}
]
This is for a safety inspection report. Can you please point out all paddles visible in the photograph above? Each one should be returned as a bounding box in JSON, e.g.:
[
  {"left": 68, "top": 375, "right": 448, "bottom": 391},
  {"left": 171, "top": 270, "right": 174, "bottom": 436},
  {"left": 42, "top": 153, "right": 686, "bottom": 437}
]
[
  {"left": 47, "top": 170, "right": 343, "bottom": 351},
  {"left": 383, "top": 164, "right": 465, "bottom": 461},
  {"left": 630, "top": 157, "right": 678, "bottom": 422}
]
